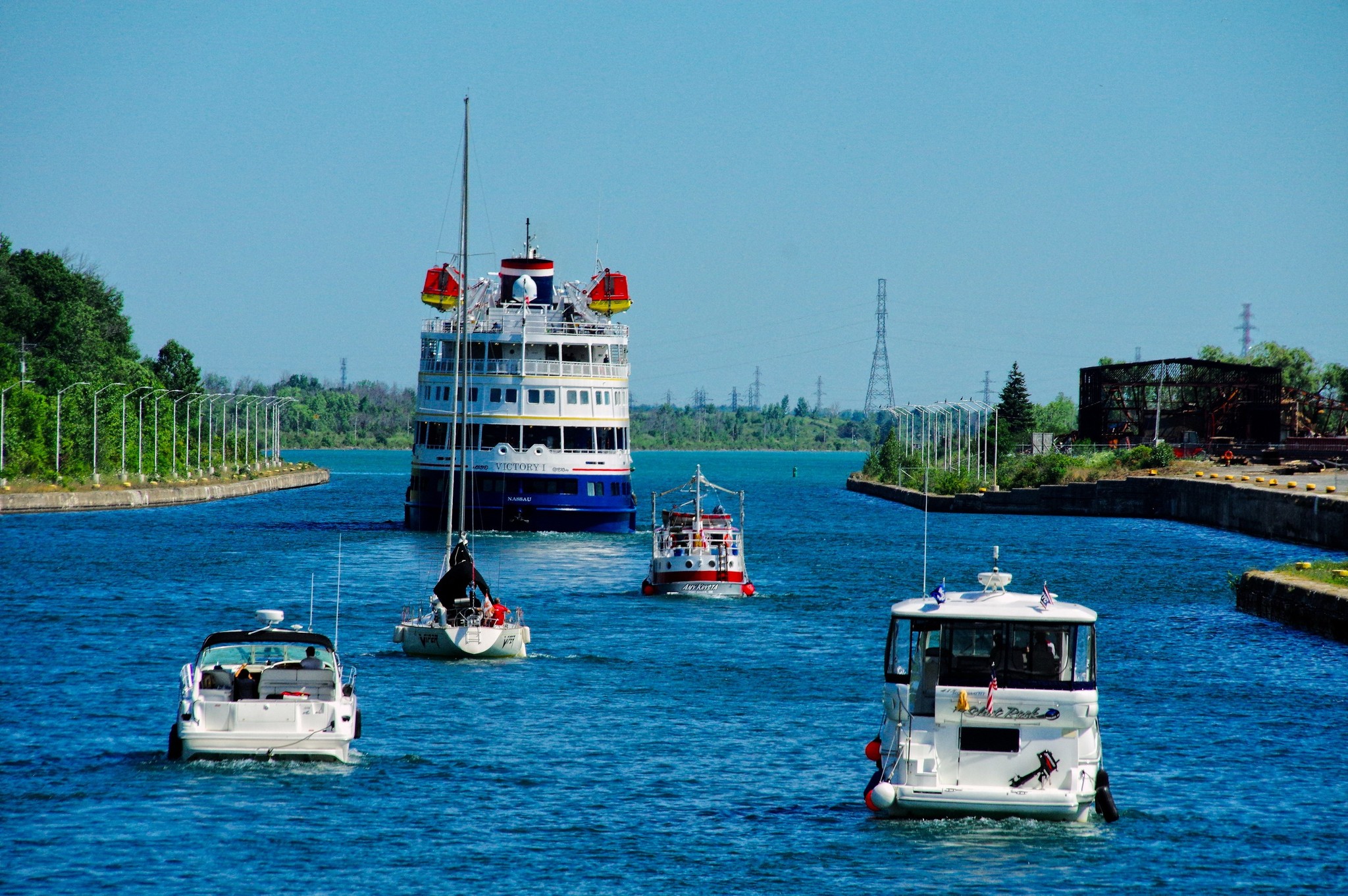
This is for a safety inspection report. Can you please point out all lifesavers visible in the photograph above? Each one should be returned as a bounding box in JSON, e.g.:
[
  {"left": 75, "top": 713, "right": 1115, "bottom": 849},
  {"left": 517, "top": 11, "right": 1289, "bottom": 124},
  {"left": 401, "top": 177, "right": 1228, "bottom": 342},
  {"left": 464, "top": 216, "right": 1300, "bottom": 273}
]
[
  {"left": 702, "top": 531, "right": 707, "bottom": 548},
  {"left": 725, "top": 534, "right": 732, "bottom": 547},
  {"left": 1224, "top": 451, "right": 1233, "bottom": 460},
  {"left": 666, "top": 534, "right": 676, "bottom": 549}
]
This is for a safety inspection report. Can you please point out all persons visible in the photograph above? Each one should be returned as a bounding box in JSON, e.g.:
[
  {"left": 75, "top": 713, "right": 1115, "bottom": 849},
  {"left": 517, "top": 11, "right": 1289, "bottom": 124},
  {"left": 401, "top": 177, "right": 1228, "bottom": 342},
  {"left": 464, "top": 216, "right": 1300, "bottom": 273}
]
[
  {"left": 483, "top": 598, "right": 511, "bottom": 627},
  {"left": 1027, "top": 631, "right": 1055, "bottom": 658},
  {"left": 296, "top": 646, "right": 325, "bottom": 670},
  {"left": 990, "top": 634, "right": 1009, "bottom": 664}
]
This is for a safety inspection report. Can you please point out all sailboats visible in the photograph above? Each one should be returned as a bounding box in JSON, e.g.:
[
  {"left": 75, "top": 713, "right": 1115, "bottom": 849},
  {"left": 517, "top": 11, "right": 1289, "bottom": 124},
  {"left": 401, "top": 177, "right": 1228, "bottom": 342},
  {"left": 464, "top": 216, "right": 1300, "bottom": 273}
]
[{"left": 392, "top": 96, "right": 534, "bottom": 660}]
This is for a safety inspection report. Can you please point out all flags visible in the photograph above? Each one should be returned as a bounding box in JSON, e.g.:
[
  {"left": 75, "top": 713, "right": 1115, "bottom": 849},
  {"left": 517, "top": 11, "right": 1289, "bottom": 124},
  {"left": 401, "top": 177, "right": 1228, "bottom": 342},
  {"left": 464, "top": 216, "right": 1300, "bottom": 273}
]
[
  {"left": 525, "top": 289, "right": 530, "bottom": 304},
  {"left": 986, "top": 667, "right": 997, "bottom": 713},
  {"left": 931, "top": 582, "right": 946, "bottom": 604},
  {"left": 1040, "top": 586, "right": 1053, "bottom": 608},
  {"left": 483, "top": 594, "right": 494, "bottom": 617},
  {"left": 701, "top": 529, "right": 706, "bottom": 549}
]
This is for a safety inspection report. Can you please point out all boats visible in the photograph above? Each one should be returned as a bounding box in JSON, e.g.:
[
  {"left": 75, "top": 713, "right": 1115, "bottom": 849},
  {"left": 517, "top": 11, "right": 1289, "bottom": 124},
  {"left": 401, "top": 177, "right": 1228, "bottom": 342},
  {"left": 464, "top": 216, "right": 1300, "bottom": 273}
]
[
  {"left": 1241, "top": 476, "right": 1250, "bottom": 481},
  {"left": 862, "top": 467, "right": 1119, "bottom": 827},
  {"left": 1210, "top": 473, "right": 1219, "bottom": 478},
  {"left": 642, "top": 464, "right": 754, "bottom": 597},
  {"left": 1255, "top": 477, "right": 1265, "bottom": 482},
  {"left": 1295, "top": 562, "right": 1312, "bottom": 570},
  {"left": 397, "top": 216, "right": 643, "bottom": 534},
  {"left": 1195, "top": 472, "right": 1203, "bottom": 477},
  {"left": 1225, "top": 475, "right": 1234, "bottom": 480},
  {"left": 168, "top": 531, "right": 362, "bottom": 763},
  {"left": 1332, "top": 569, "right": 1348, "bottom": 577},
  {"left": 1147, "top": 469, "right": 1157, "bottom": 476},
  {"left": 1269, "top": 478, "right": 1278, "bottom": 486},
  {"left": 1326, "top": 486, "right": 1336, "bottom": 492},
  {"left": 1306, "top": 483, "right": 1315, "bottom": 491},
  {"left": 1287, "top": 481, "right": 1297, "bottom": 488}
]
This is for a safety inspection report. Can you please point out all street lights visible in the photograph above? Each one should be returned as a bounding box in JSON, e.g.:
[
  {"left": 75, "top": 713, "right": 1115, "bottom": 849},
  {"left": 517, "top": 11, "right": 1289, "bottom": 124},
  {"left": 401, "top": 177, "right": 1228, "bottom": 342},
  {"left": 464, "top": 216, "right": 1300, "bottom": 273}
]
[
  {"left": 0, "top": 380, "right": 300, "bottom": 487},
  {"left": 876, "top": 399, "right": 1000, "bottom": 492}
]
